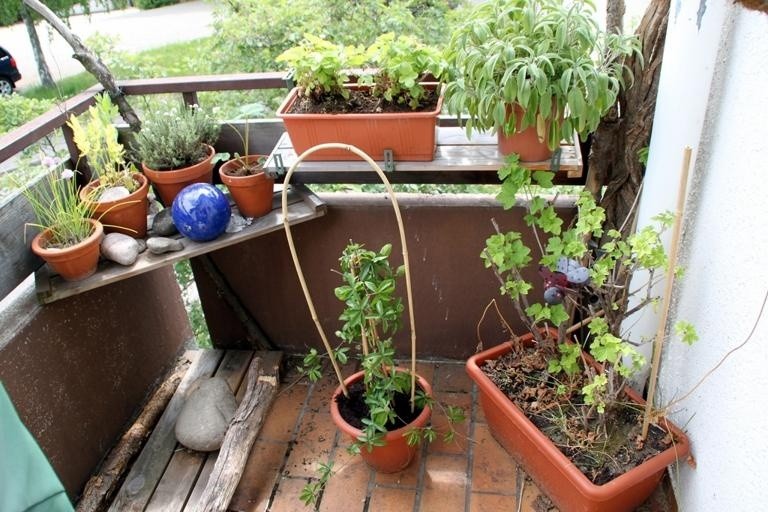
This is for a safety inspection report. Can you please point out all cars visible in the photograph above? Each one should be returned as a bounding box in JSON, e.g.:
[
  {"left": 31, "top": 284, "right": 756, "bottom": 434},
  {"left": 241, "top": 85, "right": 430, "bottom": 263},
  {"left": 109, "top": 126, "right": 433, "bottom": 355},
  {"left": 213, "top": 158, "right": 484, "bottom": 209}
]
[{"left": 0, "top": 46, "right": 21, "bottom": 93}]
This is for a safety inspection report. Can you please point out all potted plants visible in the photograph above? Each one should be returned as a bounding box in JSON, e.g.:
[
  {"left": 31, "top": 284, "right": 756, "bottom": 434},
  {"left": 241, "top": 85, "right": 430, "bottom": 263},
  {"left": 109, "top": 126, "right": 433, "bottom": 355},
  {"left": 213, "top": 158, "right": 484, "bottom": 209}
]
[
  {"left": 217, "top": 117, "right": 277, "bottom": 216},
  {"left": 0, "top": 124, "right": 142, "bottom": 283},
  {"left": 435, "top": 1, "right": 645, "bottom": 163},
  {"left": 274, "top": 27, "right": 446, "bottom": 161},
  {"left": 63, "top": 90, "right": 150, "bottom": 242},
  {"left": 466, "top": 150, "right": 699, "bottom": 512},
  {"left": 120, "top": 102, "right": 220, "bottom": 210},
  {"left": 296, "top": 238, "right": 480, "bottom": 512}
]
